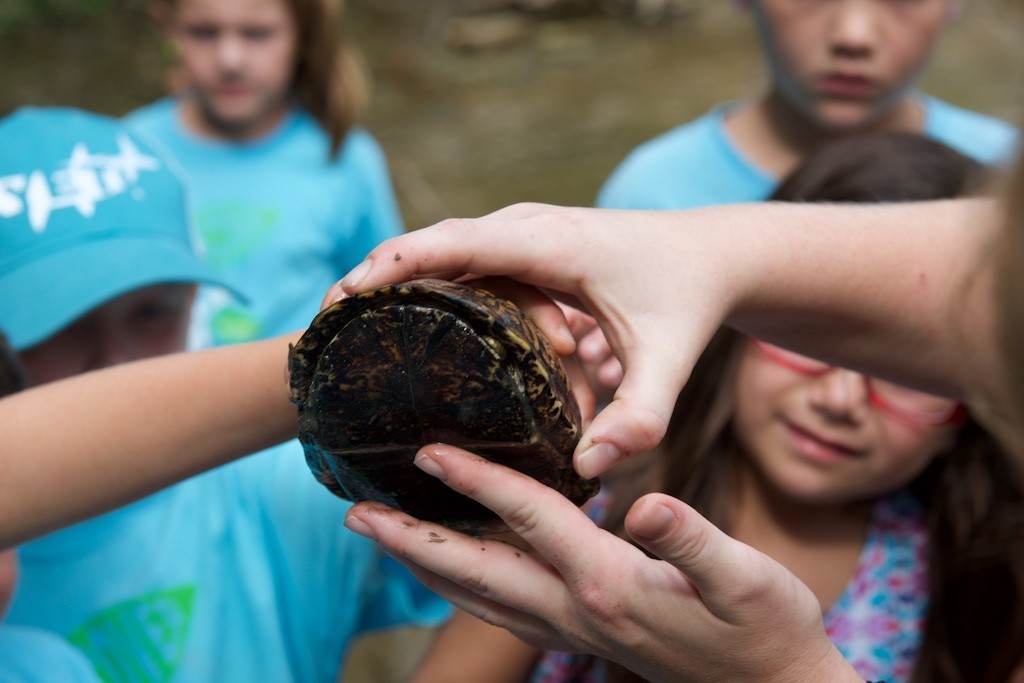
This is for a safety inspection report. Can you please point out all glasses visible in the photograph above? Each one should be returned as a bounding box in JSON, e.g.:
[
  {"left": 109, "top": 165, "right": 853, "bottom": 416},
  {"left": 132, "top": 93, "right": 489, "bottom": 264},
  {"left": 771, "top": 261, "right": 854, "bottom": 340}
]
[{"left": 755, "top": 339, "right": 965, "bottom": 432}]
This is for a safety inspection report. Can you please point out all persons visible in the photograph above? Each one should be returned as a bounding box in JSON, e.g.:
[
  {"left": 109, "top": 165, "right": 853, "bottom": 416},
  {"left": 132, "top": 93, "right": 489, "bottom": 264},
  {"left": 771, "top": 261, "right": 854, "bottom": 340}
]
[
  {"left": 0, "top": 331, "right": 99, "bottom": 683},
  {"left": 120, "top": 2, "right": 408, "bottom": 356},
  {"left": 594, "top": 1, "right": 1022, "bottom": 210},
  {"left": 1, "top": 107, "right": 454, "bottom": 683},
  {"left": 417, "top": 125, "right": 1023, "bottom": 681},
  {"left": 335, "top": 143, "right": 1024, "bottom": 683},
  {"left": 0, "top": 279, "right": 623, "bottom": 543}
]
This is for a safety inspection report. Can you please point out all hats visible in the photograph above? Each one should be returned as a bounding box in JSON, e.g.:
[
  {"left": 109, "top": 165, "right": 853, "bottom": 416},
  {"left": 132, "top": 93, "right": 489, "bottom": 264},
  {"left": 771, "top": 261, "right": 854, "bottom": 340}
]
[{"left": 0, "top": 106, "right": 252, "bottom": 354}]
